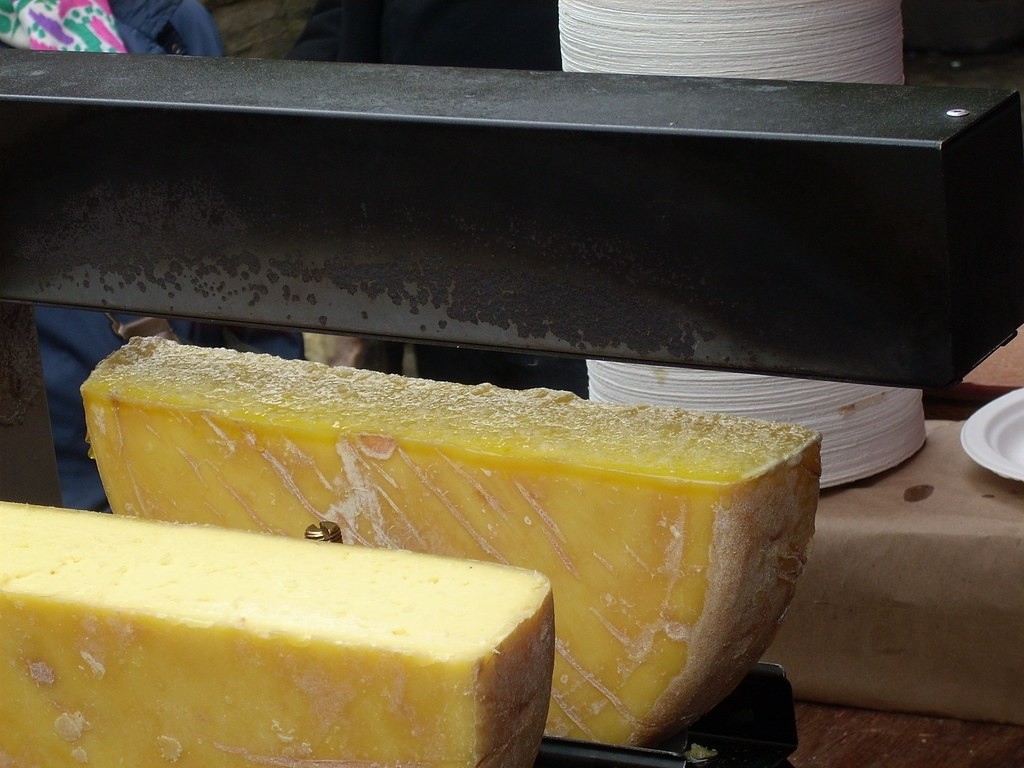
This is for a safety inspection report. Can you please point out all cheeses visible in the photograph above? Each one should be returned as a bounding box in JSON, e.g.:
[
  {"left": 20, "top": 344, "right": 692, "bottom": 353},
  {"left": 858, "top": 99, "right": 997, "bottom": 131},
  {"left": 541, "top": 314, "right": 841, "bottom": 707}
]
[{"left": 0, "top": 339, "right": 822, "bottom": 768}]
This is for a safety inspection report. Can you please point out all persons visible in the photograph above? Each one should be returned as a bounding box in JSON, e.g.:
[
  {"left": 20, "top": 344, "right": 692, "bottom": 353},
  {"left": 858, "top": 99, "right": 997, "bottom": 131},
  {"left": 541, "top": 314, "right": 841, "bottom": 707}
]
[{"left": 0, "top": 0, "right": 589, "bottom": 513}]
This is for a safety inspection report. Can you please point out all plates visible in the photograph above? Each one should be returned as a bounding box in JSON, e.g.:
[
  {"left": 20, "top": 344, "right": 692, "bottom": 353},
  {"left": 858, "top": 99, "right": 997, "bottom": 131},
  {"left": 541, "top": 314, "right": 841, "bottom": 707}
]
[
  {"left": 559, "top": 0, "right": 927, "bottom": 491},
  {"left": 959, "top": 385, "right": 1024, "bottom": 482}
]
